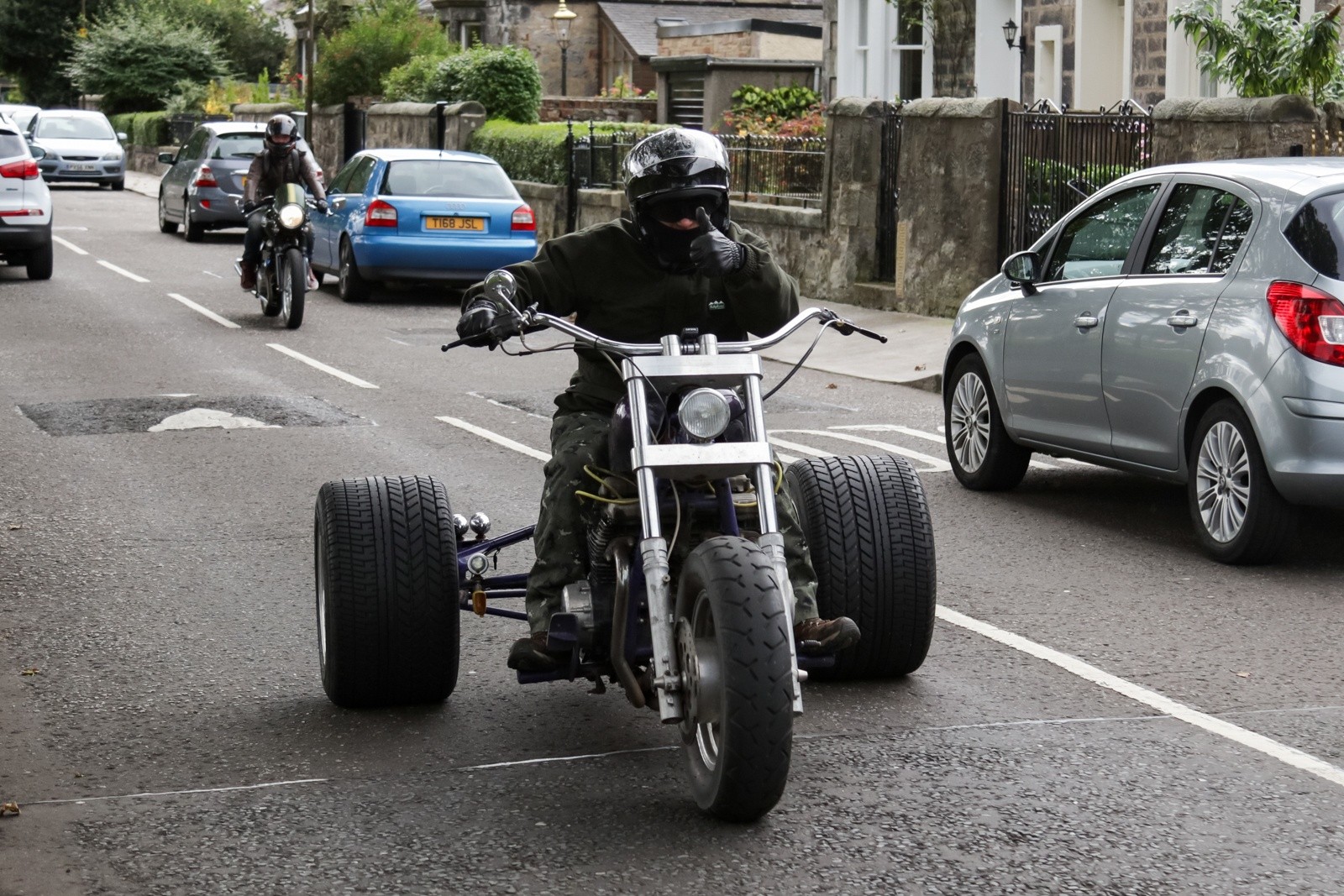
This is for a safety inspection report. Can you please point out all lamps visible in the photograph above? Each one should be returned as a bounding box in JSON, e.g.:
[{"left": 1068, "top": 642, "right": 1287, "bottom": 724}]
[{"left": 1003, "top": 18, "right": 1027, "bottom": 57}]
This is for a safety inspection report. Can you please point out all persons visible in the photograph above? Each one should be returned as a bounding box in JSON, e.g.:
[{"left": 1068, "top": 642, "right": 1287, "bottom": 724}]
[
  {"left": 239, "top": 114, "right": 329, "bottom": 292},
  {"left": 457, "top": 129, "right": 860, "bottom": 674}
]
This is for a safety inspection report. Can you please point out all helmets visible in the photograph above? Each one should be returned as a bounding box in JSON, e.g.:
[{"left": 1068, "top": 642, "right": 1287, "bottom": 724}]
[
  {"left": 263, "top": 114, "right": 297, "bottom": 157},
  {"left": 622, "top": 128, "right": 731, "bottom": 255}
]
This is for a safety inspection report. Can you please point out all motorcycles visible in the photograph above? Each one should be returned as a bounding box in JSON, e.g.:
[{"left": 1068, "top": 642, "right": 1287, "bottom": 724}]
[
  {"left": 236, "top": 182, "right": 347, "bottom": 333},
  {"left": 307, "top": 261, "right": 941, "bottom": 828}
]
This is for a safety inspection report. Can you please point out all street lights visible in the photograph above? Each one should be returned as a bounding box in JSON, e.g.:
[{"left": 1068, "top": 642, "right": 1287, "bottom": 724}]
[{"left": 548, "top": 0, "right": 577, "bottom": 98}]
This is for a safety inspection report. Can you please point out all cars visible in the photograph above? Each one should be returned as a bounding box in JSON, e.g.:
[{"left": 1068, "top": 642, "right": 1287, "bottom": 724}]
[
  {"left": 938, "top": 151, "right": 1344, "bottom": 569},
  {"left": 299, "top": 146, "right": 539, "bottom": 302},
  {"left": 22, "top": 107, "right": 129, "bottom": 191},
  {"left": 0, "top": 104, "right": 44, "bottom": 144},
  {"left": 0, "top": 109, "right": 57, "bottom": 282},
  {"left": 155, "top": 121, "right": 325, "bottom": 244}
]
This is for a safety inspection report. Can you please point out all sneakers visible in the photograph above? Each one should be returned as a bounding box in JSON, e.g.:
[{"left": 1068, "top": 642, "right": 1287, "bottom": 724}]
[
  {"left": 797, "top": 617, "right": 862, "bottom": 664},
  {"left": 304, "top": 269, "right": 320, "bottom": 291},
  {"left": 241, "top": 265, "right": 256, "bottom": 289},
  {"left": 509, "top": 632, "right": 554, "bottom": 673}
]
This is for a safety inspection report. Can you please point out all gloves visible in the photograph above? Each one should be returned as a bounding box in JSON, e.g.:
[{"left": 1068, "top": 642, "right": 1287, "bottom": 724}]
[
  {"left": 687, "top": 206, "right": 743, "bottom": 276},
  {"left": 457, "top": 308, "right": 498, "bottom": 352},
  {"left": 245, "top": 200, "right": 257, "bottom": 212},
  {"left": 316, "top": 200, "right": 329, "bottom": 214}
]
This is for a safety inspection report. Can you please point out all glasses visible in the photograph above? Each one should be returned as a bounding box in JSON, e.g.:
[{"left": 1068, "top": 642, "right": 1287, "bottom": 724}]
[{"left": 656, "top": 196, "right": 720, "bottom": 222}]
